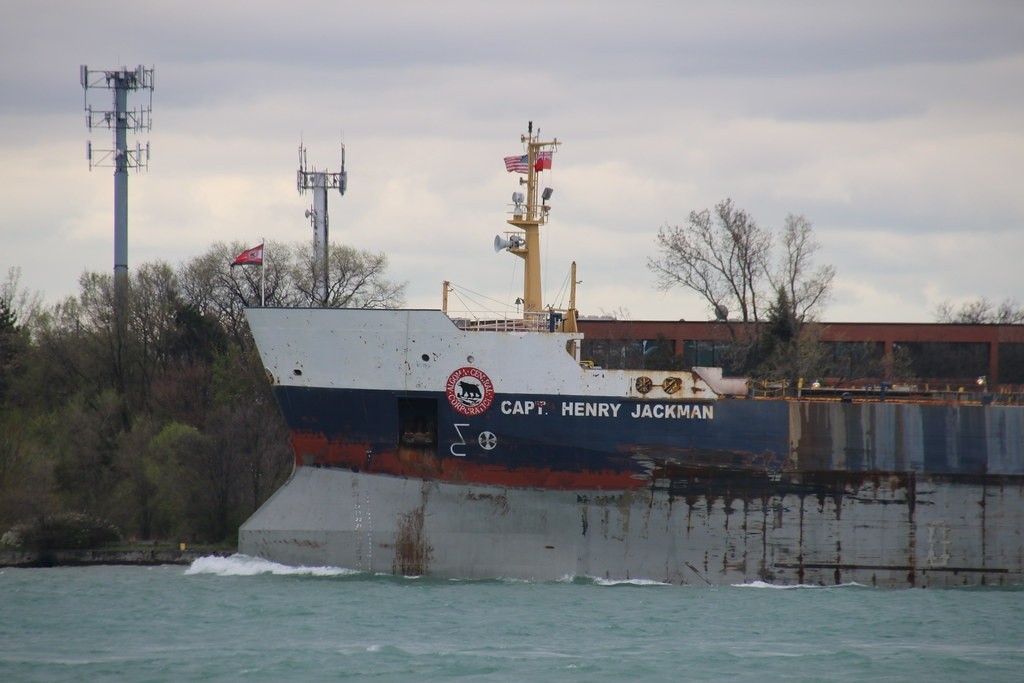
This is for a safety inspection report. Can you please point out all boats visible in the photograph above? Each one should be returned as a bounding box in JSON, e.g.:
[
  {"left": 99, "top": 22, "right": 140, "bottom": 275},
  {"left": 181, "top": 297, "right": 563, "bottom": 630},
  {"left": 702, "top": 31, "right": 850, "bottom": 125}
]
[{"left": 237, "top": 121, "right": 1023, "bottom": 589}]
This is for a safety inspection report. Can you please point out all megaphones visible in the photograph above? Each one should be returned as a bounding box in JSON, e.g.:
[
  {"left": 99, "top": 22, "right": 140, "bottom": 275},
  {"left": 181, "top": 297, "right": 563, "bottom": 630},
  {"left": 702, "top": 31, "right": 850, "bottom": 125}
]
[{"left": 494, "top": 236, "right": 516, "bottom": 252}]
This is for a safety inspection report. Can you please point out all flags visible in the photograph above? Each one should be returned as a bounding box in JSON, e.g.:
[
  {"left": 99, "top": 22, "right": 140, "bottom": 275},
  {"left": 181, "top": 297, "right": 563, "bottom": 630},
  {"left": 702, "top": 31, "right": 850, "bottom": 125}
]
[
  {"left": 502, "top": 150, "right": 554, "bottom": 174},
  {"left": 230, "top": 245, "right": 263, "bottom": 265}
]
